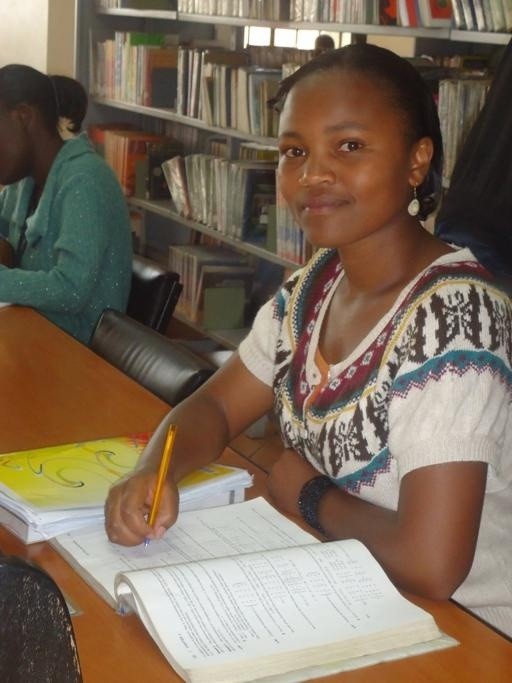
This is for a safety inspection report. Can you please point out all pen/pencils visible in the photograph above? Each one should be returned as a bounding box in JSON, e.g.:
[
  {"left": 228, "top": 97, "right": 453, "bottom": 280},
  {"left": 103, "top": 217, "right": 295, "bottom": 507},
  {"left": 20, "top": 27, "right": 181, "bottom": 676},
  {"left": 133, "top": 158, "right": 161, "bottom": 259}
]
[{"left": 145, "top": 423, "right": 177, "bottom": 546}]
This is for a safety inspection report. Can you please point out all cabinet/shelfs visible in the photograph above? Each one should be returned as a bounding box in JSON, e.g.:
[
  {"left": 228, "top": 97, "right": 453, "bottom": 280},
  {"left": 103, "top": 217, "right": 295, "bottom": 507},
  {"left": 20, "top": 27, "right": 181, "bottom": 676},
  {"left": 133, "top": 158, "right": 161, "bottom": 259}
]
[{"left": 74, "top": 0, "right": 512, "bottom": 440}]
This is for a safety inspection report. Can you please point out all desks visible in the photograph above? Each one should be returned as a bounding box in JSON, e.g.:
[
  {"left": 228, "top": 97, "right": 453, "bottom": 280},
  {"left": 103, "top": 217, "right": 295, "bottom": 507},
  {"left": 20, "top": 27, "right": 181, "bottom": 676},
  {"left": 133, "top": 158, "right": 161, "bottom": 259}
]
[{"left": 0, "top": 303, "right": 512, "bottom": 683}]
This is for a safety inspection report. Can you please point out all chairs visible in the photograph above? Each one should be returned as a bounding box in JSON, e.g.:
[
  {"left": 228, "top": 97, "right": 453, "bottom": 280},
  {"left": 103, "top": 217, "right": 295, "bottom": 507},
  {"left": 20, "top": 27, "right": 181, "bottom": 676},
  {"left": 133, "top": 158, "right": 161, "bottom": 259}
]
[{"left": 90, "top": 252, "right": 220, "bottom": 408}]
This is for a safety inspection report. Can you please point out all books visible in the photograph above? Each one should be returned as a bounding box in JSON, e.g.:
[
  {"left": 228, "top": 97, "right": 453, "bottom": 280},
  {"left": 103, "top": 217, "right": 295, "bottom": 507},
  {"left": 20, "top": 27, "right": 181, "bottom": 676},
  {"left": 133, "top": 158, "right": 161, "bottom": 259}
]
[
  {"left": 168, "top": 242, "right": 255, "bottom": 323},
  {"left": 92, "top": 115, "right": 319, "bottom": 265},
  {"left": 177, "top": 0, "right": 511, "bottom": 34},
  {"left": 404, "top": 56, "right": 492, "bottom": 179},
  {"left": 95, "top": 31, "right": 321, "bottom": 139},
  {"left": 96, "top": 0, "right": 173, "bottom": 11},
  {"left": 0, "top": 433, "right": 254, "bottom": 545},
  {"left": 50, "top": 495, "right": 461, "bottom": 683}
]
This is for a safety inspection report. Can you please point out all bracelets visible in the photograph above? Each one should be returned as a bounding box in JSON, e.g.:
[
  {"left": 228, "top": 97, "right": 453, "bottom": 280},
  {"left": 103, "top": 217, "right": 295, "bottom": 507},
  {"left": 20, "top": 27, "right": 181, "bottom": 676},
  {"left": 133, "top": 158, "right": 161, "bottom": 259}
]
[{"left": 298, "top": 474, "right": 336, "bottom": 534}]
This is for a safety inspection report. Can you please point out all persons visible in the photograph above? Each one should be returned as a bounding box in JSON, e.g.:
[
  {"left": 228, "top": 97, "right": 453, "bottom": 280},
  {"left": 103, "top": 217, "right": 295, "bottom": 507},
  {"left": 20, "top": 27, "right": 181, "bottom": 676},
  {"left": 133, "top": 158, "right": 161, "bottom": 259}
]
[
  {"left": 104, "top": 42, "right": 512, "bottom": 641},
  {"left": 0, "top": 64, "right": 134, "bottom": 348}
]
